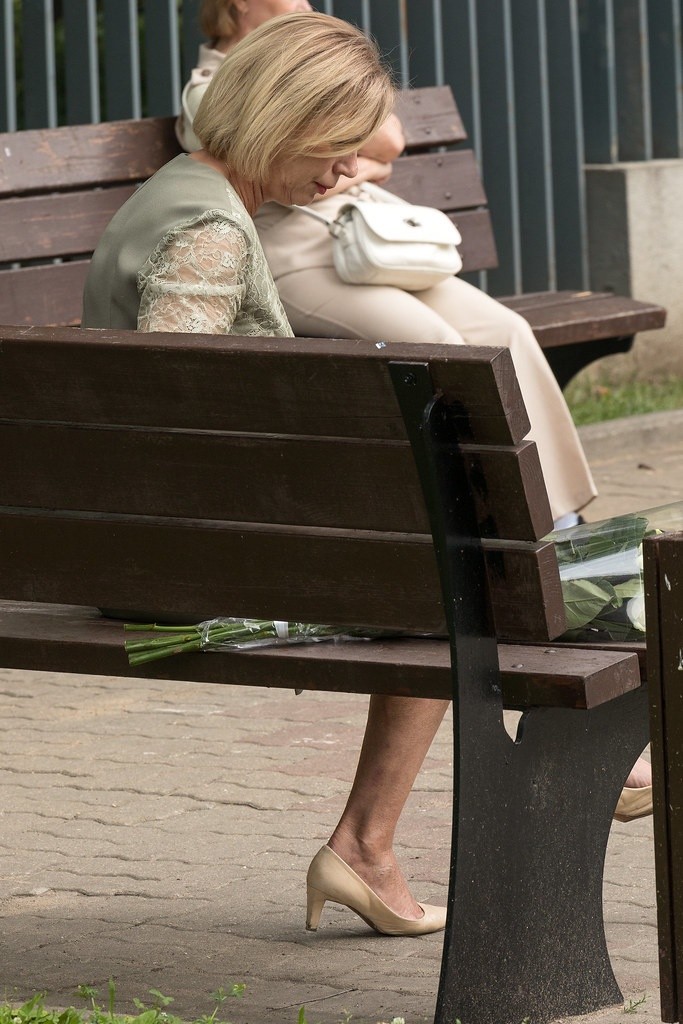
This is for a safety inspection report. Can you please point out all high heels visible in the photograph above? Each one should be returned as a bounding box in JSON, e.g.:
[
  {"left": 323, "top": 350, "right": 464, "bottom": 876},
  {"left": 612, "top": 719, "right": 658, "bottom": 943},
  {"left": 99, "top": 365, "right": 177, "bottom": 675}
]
[
  {"left": 614, "top": 784, "right": 654, "bottom": 823},
  {"left": 306, "top": 845, "right": 447, "bottom": 937}
]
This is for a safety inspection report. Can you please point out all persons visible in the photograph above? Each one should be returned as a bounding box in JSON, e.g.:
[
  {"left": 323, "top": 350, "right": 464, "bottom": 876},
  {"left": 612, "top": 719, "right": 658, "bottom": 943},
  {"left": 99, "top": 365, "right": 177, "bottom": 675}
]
[
  {"left": 83, "top": 12, "right": 651, "bottom": 930},
  {"left": 175, "top": 0, "right": 597, "bottom": 533}
]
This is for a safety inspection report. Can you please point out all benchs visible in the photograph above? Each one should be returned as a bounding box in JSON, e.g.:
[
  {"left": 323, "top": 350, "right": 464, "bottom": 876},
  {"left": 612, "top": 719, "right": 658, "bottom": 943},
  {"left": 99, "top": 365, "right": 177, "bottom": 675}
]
[
  {"left": 0, "top": 83, "right": 667, "bottom": 394},
  {"left": 0, "top": 322, "right": 683, "bottom": 1024}
]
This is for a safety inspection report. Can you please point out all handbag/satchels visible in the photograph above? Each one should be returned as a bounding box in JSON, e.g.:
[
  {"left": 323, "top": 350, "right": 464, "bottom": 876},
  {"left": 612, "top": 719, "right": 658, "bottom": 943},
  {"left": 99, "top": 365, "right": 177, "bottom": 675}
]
[{"left": 330, "top": 200, "right": 462, "bottom": 293}]
